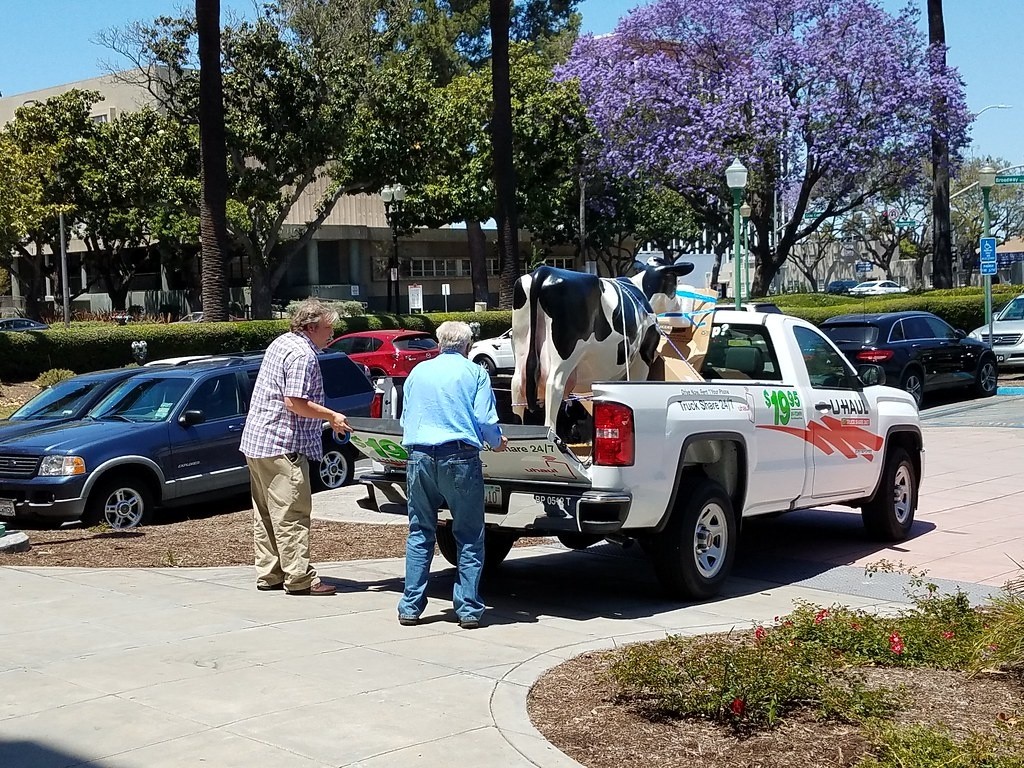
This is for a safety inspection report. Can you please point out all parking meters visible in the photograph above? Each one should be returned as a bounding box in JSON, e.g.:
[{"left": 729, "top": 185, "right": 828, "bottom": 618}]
[{"left": 131, "top": 341, "right": 148, "bottom": 366}]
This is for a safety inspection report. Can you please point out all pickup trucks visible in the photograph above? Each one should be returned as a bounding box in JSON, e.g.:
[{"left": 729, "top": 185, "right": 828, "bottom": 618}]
[{"left": 343, "top": 309, "right": 926, "bottom": 603}]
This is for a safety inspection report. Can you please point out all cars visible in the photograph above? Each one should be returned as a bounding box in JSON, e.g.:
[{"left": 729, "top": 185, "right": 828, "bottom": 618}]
[
  {"left": 967, "top": 293, "right": 1024, "bottom": 368},
  {"left": 848, "top": 280, "right": 909, "bottom": 296},
  {"left": 711, "top": 302, "right": 783, "bottom": 351},
  {"left": 168, "top": 312, "right": 247, "bottom": 324},
  {"left": 823, "top": 279, "right": 859, "bottom": 295},
  {"left": 0, "top": 317, "right": 51, "bottom": 333},
  {"left": 0, "top": 327, "right": 439, "bottom": 530},
  {"left": 466, "top": 327, "right": 517, "bottom": 377},
  {"left": 801, "top": 310, "right": 999, "bottom": 411}
]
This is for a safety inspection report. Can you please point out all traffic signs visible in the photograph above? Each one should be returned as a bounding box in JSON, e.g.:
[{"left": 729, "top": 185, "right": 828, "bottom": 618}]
[{"left": 804, "top": 212, "right": 822, "bottom": 219}]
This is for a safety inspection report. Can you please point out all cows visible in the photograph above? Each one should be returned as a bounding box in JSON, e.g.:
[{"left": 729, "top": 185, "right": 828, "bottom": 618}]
[{"left": 510, "top": 256, "right": 694, "bottom": 435}]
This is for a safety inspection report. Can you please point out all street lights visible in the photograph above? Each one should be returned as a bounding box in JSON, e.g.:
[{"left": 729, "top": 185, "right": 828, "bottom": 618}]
[
  {"left": 977, "top": 163, "right": 997, "bottom": 325},
  {"left": 380, "top": 182, "right": 405, "bottom": 316},
  {"left": 724, "top": 158, "right": 749, "bottom": 312}
]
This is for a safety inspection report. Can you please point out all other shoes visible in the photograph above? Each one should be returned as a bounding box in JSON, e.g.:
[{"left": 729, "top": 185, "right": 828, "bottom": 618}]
[
  {"left": 460, "top": 619, "right": 479, "bottom": 627},
  {"left": 257, "top": 580, "right": 285, "bottom": 590},
  {"left": 290, "top": 581, "right": 337, "bottom": 595},
  {"left": 398, "top": 614, "right": 419, "bottom": 625}
]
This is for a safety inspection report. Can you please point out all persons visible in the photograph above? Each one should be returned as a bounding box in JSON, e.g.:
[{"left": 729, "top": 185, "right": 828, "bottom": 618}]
[
  {"left": 398, "top": 321, "right": 508, "bottom": 626},
  {"left": 239, "top": 297, "right": 354, "bottom": 595}
]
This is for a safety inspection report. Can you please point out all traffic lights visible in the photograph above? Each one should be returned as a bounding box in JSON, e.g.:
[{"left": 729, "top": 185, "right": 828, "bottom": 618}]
[{"left": 881, "top": 210, "right": 889, "bottom": 227}]
